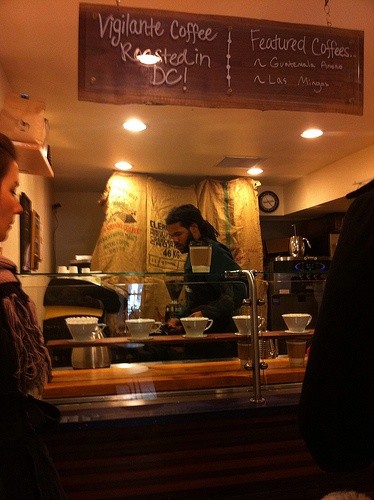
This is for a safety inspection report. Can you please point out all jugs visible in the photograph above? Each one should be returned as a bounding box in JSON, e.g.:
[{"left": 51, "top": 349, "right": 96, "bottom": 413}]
[{"left": 72, "top": 323, "right": 110, "bottom": 369}]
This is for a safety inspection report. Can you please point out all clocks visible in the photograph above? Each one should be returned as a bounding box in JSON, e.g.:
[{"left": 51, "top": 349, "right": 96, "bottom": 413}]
[{"left": 257, "top": 190, "right": 279, "bottom": 213}]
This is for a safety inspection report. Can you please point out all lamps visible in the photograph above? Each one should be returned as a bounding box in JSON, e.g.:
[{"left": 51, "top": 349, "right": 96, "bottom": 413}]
[{"left": 52, "top": 202, "right": 62, "bottom": 210}]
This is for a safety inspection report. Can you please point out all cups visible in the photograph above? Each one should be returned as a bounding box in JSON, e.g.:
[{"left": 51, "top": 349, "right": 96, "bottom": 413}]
[
  {"left": 231, "top": 315, "right": 265, "bottom": 335},
  {"left": 289, "top": 236, "right": 305, "bottom": 259},
  {"left": 125, "top": 319, "right": 162, "bottom": 338},
  {"left": 236, "top": 341, "right": 253, "bottom": 370},
  {"left": 286, "top": 339, "right": 306, "bottom": 367},
  {"left": 180, "top": 317, "right": 213, "bottom": 338},
  {"left": 189, "top": 241, "right": 211, "bottom": 273},
  {"left": 64, "top": 317, "right": 105, "bottom": 341},
  {"left": 282, "top": 314, "right": 311, "bottom": 332}
]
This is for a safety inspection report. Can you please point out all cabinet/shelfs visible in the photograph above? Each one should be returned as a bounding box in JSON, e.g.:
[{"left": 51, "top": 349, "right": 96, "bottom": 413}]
[{"left": 16, "top": 267, "right": 330, "bottom": 399}]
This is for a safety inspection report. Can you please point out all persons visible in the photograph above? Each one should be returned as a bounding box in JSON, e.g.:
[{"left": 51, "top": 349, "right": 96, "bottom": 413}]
[
  {"left": 0, "top": 132, "right": 53, "bottom": 500},
  {"left": 166, "top": 204, "right": 248, "bottom": 358},
  {"left": 300, "top": 179, "right": 374, "bottom": 466}
]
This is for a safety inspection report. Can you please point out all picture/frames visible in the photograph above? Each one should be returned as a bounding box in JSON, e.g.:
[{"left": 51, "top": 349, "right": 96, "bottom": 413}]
[{"left": 18, "top": 191, "right": 41, "bottom": 273}]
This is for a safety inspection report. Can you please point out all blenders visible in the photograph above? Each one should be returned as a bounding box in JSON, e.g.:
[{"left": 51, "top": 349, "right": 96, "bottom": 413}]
[{"left": 162, "top": 271, "right": 184, "bottom": 336}]
